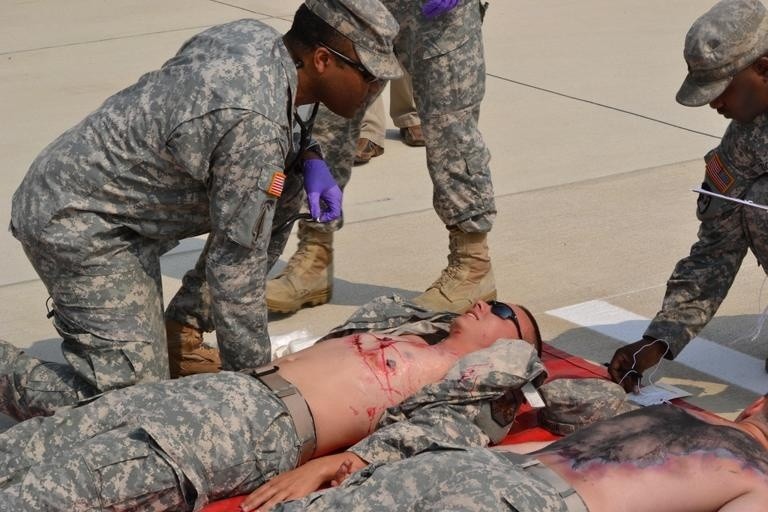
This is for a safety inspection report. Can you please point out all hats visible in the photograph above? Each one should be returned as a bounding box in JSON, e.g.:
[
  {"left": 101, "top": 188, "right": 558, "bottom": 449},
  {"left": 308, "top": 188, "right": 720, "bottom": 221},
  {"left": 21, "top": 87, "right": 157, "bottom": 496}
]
[
  {"left": 305, "top": 0, "right": 405, "bottom": 79},
  {"left": 676, "top": 0, "right": 768, "bottom": 106},
  {"left": 540, "top": 377, "right": 640, "bottom": 436}
]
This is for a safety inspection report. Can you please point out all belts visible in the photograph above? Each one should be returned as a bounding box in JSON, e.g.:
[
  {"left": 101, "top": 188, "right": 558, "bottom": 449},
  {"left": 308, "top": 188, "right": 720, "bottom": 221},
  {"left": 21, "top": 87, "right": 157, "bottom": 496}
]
[
  {"left": 251, "top": 366, "right": 317, "bottom": 466},
  {"left": 513, "top": 454, "right": 588, "bottom": 512}
]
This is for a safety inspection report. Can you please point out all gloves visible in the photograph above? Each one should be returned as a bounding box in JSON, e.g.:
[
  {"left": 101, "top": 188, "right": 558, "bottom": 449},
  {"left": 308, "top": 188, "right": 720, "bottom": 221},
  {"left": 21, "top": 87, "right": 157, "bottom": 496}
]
[
  {"left": 304, "top": 159, "right": 342, "bottom": 223},
  {"left": 423, "top": 0, "right": 460, "bottom": 18}
]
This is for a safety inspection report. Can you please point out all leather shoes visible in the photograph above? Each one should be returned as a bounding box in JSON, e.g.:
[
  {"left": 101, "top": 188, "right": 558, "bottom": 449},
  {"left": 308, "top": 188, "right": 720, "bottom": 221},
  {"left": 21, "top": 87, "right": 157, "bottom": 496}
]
[
  {"left": 400, "top": 126, "right": 425, "bottom": 146},
  {"left": 354, "top": 138, "right": 384, "bottom": 162}
]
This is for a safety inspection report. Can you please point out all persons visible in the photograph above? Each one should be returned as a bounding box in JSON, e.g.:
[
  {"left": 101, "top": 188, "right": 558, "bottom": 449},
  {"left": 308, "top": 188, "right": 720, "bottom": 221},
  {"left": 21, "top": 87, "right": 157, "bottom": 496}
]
[
  {"left": 260, "top": 0, "right": 497, "bottom": 321},
  {"left": 1, "top": 0, "right": 406, "bottom": 420},
  {"left": 349, "top": 58, "right": 426, "bottom": 164},
  {"left": 1, "top": 294, "right": 543, "bottom": 512},
  {"left": 609, "top": 0, "right": 768, "bottom": 393},
  {"left": 265, "top": 382, "right": 768, "bottom": 512}
]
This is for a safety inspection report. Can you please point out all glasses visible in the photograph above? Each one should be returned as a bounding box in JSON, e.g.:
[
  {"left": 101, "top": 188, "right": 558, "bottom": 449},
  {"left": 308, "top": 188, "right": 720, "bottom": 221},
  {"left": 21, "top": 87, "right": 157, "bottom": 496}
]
[
  {"left": 320, "top": 42, "right": 377, "bottom": 84},
  {"left": 487, "top": 299, "right": 523, "bottom": 339}
]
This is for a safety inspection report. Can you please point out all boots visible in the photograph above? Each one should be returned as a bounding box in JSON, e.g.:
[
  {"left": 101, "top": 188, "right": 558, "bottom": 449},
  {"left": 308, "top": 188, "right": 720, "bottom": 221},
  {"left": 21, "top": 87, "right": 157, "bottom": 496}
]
[
  {"left": 266, "top": 228, "right": 333, "bottom": 314},
  {"left": 412, "top": 231, "right": 497, "bottom": 314},
  {"left": 163, "top": 316, "right": 222, "bottom": 375}
]
